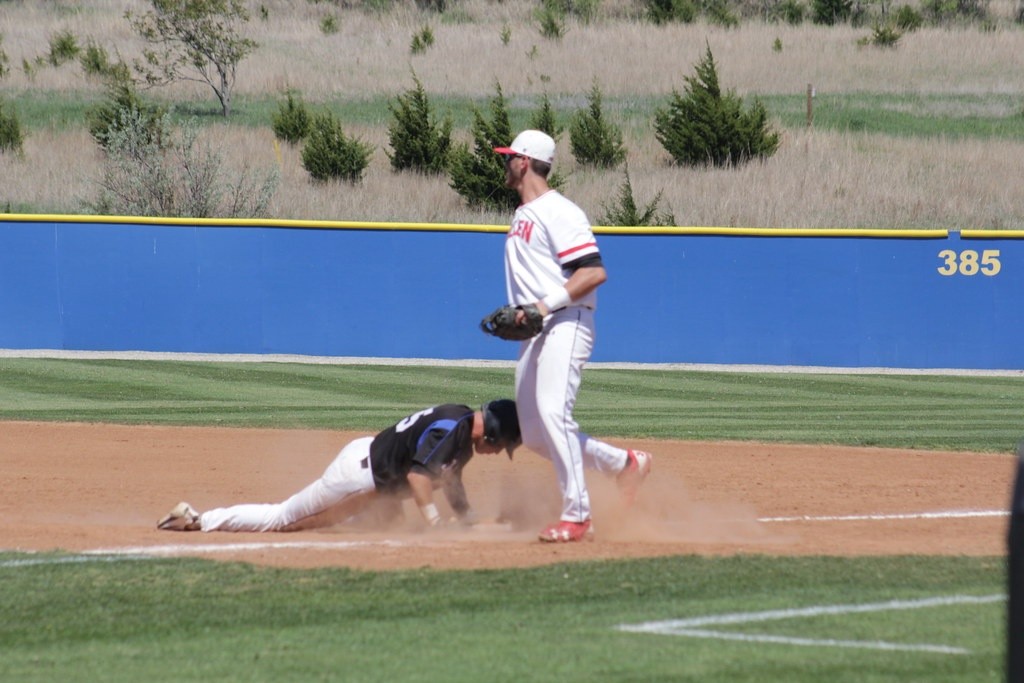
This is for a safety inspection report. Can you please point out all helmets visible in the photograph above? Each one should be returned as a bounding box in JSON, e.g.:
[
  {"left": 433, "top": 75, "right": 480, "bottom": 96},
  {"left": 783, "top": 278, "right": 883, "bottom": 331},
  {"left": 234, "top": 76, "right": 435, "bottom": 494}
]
[{"left": 481, "top": 399, "right": 522, "bottom": 461}]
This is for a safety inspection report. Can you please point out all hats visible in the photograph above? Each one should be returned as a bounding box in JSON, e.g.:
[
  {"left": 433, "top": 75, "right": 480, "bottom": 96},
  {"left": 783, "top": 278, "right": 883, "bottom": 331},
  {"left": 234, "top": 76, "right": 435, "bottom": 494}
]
[{"left": 493, "top": 130, "right": 556, "bottom": 164}]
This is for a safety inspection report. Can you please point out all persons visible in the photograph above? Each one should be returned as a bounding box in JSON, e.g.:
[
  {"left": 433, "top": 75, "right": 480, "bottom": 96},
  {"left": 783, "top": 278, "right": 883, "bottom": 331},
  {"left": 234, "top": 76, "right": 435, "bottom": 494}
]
[
  {"left": 156, "top": 399, "right": 523, "bottom": 540},
  {"left": 479, "top": 128, "right": 654, "bottom": 545}
]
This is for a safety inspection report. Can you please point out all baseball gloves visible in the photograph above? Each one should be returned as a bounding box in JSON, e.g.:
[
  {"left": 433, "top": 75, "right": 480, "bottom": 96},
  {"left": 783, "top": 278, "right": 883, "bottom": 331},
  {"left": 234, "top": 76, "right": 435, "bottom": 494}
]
[{"left": 480, "top": 303, "right": 545, "bottom": 343}]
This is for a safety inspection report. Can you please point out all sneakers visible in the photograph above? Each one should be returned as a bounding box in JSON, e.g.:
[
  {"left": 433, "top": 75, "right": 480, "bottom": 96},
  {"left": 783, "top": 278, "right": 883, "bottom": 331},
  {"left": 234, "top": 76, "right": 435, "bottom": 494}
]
[
  {"left": 614, "top": 450, "right": 653, "bottom": 509},
  {"left": 538, "top": 516, "right": 594, "bottom": 543},
  {"left": 157, "top": 502, "right": 200, "bottom": 531}
]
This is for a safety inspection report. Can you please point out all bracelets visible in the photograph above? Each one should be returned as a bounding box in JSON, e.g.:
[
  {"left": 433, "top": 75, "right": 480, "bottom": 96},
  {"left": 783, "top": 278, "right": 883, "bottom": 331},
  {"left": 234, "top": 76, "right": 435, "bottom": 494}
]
[
  {"left": 420, "top": 502, "right": 440, "bottom": 522},
  {"left": 540, "top": 286, "right": 570, "bottom": 312}
]
